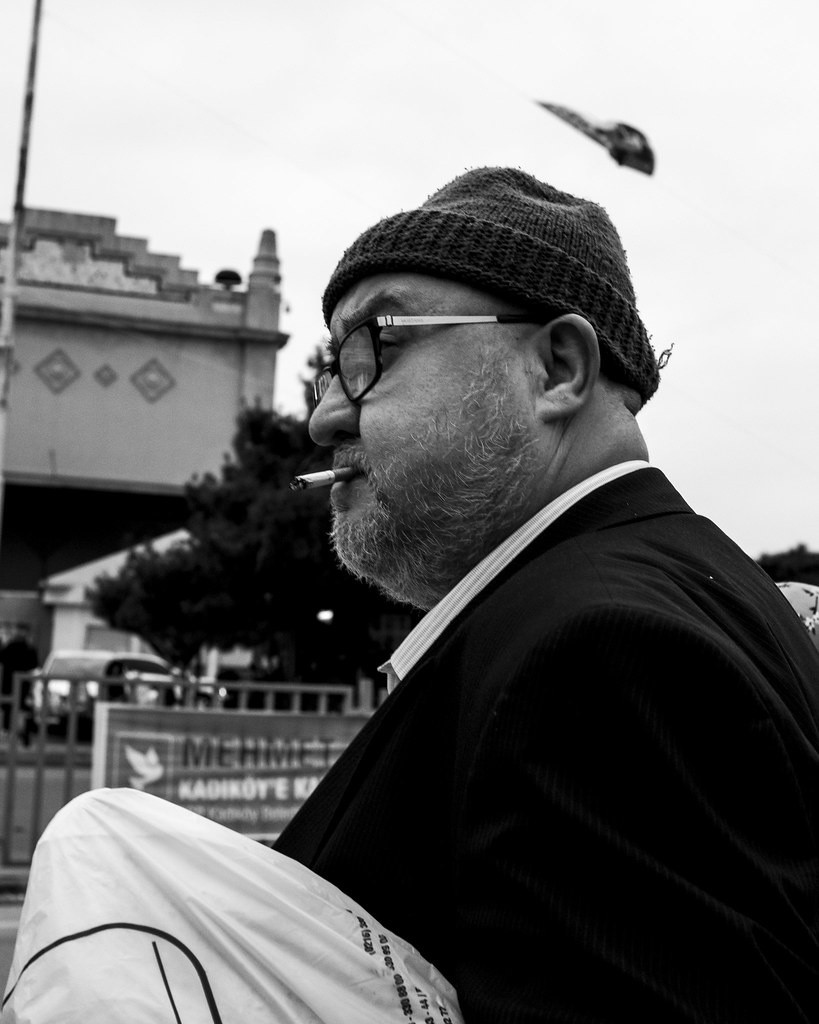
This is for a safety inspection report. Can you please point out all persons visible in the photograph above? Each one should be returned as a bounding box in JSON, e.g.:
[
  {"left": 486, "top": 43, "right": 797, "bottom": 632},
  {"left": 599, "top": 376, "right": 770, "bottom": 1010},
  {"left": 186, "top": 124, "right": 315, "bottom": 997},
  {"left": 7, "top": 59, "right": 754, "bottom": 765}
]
[
  {"left": 272, "top": 164, "right": 819, "bottom": 1024},
  {"left": 0, "top": 621, "right": 41, "bottom": 748}
]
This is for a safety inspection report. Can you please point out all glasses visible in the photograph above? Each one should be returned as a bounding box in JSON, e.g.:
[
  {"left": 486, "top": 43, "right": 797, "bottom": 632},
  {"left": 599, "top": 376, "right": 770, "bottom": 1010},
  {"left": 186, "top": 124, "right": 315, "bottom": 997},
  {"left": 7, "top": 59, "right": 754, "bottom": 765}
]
[{"left": 312, "top": 312, "right": 559, "bottom": 408}]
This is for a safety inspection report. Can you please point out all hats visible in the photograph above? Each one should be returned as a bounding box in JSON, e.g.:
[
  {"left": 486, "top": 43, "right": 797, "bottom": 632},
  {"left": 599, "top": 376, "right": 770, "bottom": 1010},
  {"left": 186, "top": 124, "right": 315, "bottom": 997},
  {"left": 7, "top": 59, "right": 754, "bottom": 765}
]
[{"left": 322, "top": 166, "right": 660, "bottom": 411}]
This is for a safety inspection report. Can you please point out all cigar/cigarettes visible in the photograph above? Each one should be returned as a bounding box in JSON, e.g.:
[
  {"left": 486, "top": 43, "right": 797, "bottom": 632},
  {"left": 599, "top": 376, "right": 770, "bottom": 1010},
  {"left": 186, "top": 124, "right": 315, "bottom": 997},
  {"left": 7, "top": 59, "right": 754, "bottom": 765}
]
[{"left": 290, "top": 466, "right": 357, "bottom": 491}]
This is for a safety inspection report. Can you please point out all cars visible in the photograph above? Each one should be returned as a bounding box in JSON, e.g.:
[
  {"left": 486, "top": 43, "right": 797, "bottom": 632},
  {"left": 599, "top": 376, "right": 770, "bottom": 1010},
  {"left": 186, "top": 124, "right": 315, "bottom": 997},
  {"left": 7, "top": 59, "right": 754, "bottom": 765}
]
[{"left": 25, "top": 649, "right": 212, "bottom": 743}]
